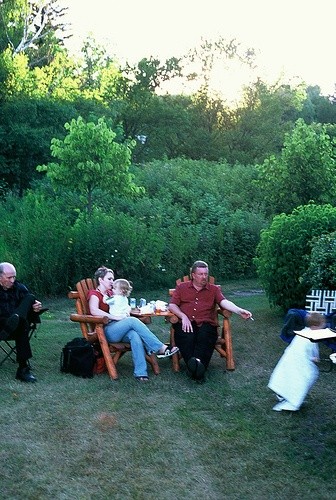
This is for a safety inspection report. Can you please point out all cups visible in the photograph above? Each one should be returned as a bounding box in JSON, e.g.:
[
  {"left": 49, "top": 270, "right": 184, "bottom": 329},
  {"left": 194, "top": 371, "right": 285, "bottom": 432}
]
[{"left": 155, "top": 303, "right": 162, "bottom": 314}]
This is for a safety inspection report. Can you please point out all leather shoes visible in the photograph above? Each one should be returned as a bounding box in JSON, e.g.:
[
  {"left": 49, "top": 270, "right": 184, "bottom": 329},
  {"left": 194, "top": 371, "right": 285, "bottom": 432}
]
[
  {"left": 0, "top": 313, "right": 18, "bottom": 341},
  {"left": 15, "top": 367, "right": 36, "bottom": 383}
]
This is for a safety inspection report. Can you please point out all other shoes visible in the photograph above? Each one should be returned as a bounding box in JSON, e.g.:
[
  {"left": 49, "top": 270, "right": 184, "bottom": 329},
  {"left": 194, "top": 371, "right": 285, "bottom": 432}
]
[
  {"left": 186, "top": 357, "right": 198, "bottom": 379},
  {"left": 194, "top": 363, "right": 205, "bottom": 384}
]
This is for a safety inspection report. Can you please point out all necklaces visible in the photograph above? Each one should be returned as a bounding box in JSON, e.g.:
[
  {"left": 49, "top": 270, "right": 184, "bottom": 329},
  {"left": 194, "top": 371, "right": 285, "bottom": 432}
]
[{"left": 96, "top": 287, "right": 102, "bottom": 293}]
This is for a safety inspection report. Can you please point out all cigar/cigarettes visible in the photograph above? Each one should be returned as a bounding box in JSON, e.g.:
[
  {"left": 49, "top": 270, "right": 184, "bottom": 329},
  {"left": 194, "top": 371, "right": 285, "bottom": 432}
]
[{"left": 248, "top": 314, "right": 255, "bottom": 322}]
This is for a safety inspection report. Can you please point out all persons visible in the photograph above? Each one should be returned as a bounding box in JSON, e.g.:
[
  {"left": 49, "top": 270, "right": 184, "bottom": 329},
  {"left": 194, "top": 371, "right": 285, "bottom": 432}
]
[
  {"left": 168, "top": 261, "right": 252, "bottom": 385},
  {"left": 0, "top": 262, "right": 42, "bottom": 384},
  {"left": 87, "top": 279, "right": 133, "bottom": 335},
  {"left": 267, "top": 313, "right": 326, "bottom": 411},
  {"left": 86, "top": 267, "right": 179, "bottom": 384}
]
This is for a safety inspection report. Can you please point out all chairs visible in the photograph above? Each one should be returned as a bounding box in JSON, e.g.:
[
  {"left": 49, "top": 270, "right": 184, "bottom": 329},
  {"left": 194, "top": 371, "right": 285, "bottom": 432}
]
[
  {"left": 68, "top": 278, "right": 160, "bottom": 381},
  {"left": 165, "top": 268, "right": 235, "bottom": 373}
]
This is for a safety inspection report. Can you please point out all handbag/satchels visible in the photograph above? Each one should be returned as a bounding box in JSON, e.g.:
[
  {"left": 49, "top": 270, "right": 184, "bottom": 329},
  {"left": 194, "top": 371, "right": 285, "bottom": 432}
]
[{"left": 61, "top": 337, "right": 98, "bottom": 379}]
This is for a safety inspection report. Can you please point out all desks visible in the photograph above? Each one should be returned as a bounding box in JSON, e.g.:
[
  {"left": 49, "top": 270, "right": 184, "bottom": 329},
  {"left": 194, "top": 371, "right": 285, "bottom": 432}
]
[
  {"left": 130, "top": 308, "right": 174, "bottom": 317},
  {"left": 0, "top": 308, "right": 49, "bottom": 370}
]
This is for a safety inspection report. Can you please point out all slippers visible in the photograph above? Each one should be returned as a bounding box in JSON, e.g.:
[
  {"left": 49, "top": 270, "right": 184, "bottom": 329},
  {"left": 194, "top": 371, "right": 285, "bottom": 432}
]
[
  {"left": 156, "top": 345, "right": 180, "bottom": 360},
  {"left": 137, "top": 376, "right": 148, "bottom": 382}
]
